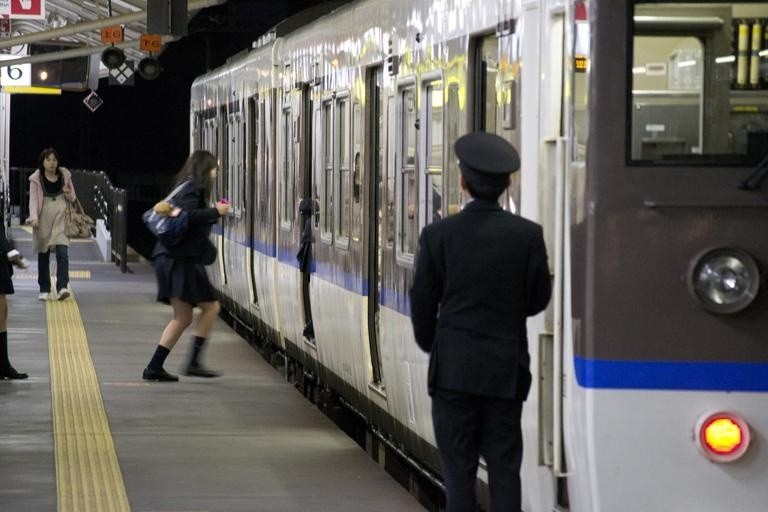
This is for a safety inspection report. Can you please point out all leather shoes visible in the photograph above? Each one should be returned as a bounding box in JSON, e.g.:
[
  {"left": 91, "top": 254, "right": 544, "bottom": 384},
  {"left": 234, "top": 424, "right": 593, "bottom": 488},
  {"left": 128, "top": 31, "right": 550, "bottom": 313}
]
[
  {"left": 142, "top": 366, "right": 178, "bottom": 382},
  {"left": 180, "top": 363, "right": 223, "bottom": 378}
]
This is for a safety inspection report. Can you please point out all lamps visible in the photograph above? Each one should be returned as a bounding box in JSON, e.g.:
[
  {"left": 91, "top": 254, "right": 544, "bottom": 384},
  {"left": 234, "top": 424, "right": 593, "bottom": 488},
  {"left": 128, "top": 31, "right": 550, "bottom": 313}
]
[
  {"left": 138, "top": 51, "right": 162, "bottom": 81},
  {"left": 101, "top": 0, "right": 124, "bottom": 70}
]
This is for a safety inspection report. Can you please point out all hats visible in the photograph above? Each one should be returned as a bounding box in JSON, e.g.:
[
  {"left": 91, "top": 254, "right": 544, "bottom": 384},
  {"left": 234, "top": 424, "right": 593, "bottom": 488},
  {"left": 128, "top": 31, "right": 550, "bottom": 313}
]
[{"left": 453, "top": 131, "right": 520, "bottom": 181}]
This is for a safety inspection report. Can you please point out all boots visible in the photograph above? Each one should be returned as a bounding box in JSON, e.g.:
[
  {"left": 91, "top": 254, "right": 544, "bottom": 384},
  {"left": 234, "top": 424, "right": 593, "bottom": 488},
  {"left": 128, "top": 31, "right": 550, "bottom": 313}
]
[{"left": 0, "top": 331, "right": 28, "bottom": 380}]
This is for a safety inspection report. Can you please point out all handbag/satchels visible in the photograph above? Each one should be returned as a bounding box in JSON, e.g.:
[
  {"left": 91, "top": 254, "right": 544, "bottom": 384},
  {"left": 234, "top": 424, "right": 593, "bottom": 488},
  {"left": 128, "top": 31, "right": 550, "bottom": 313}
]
[
  {"left": 142, "top": 199, "right": 217, "bottom": 265},
  {"left": 64, "top": 196, "right": 94, "bottom": 239}
]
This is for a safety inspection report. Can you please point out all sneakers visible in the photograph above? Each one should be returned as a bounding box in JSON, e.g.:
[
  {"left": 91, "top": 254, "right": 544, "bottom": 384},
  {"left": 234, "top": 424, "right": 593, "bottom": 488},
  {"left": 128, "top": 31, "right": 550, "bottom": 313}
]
[
  {"left": 57, "top": 287, "right": 70, "bottom": 301},
  {"left": 39, "top": 292, "right": 50, "bottom": 301}
]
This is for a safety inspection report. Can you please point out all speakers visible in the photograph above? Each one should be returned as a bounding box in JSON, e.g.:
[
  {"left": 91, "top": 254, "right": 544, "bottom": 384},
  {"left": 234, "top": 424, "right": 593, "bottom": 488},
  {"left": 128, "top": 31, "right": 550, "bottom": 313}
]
[{"left": 147, "top": 0, "right": 188, "bottom": 36}]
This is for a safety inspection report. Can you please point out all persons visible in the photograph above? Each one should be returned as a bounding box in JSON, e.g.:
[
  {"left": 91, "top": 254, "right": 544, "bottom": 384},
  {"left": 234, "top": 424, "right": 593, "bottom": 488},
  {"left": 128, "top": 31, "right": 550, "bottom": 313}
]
[
  {"left": 1, "top": 180, "right": 29, "bottom": 380},
  {"left": 24, "top": 146, "right": 77, "bottom": 301},
  {"left": 140, "top": 148, "right": 232, "bottom": 382},
  {"left": 405, "top": 130, "right": 554, "bottom": 512},
  {"left": 351, "top": 150, "right": 360, "bottom": 204},
  {"left": 295, "top": 194, "right": 314, "bottom": 339},
  {"left": 406, "top": 155, "right": 443, "bottom": 255}
]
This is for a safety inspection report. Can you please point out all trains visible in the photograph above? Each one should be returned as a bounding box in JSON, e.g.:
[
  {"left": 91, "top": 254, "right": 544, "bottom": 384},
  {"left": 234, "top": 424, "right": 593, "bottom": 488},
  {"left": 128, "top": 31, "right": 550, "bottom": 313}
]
[{"left": 183, "top": 0, "right": 767, "bottom": 511}]
